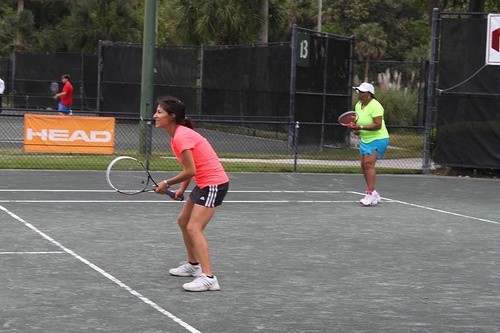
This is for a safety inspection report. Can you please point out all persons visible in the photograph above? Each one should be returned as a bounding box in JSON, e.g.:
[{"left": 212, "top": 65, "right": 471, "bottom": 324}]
[
  {"left": 0, "top": 77, "right": 5, "bottom": 113},
  {"left": 53, "top": 74, "right": 74, "bottom": 117},
  {"left": 351, "top": 81, "right": 391, "bottom": 207},
  {"left": 153, "top": 96, "right": 230, "bottom": 292},
  {"left": 50, "top": 80, "right": 59, "bottom": 98}
]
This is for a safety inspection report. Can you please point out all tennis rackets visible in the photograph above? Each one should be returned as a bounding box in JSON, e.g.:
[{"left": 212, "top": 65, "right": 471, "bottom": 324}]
[
  {"left": 338, "top": 110, "right": 359, "bottom": 137},
  {"left": 106, "top": 156, "right": 185, "bottom": 202}
]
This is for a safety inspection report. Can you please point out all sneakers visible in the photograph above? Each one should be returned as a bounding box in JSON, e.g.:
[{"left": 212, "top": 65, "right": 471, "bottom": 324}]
[
  {"left": 183, "top": 273, "right": 220, "bottom": 293},
  {"left": 168, "top": 262, "right": 202, "bottom": 277},
  {"left": 360, "top": 188, "right": 381, "bottom": 206}
]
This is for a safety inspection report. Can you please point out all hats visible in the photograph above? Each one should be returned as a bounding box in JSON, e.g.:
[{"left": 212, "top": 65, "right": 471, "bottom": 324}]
[{"left": 351, "top": 82, "right": 374, "bottom": 95}]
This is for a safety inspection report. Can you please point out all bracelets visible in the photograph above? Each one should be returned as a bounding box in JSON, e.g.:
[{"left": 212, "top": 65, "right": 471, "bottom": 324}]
[
  {"left": 163, "top": 180, "right": 170, "bottom": 189},
  {"left": 359, "top": 126, "right": 363, "bottom": 130}
]
